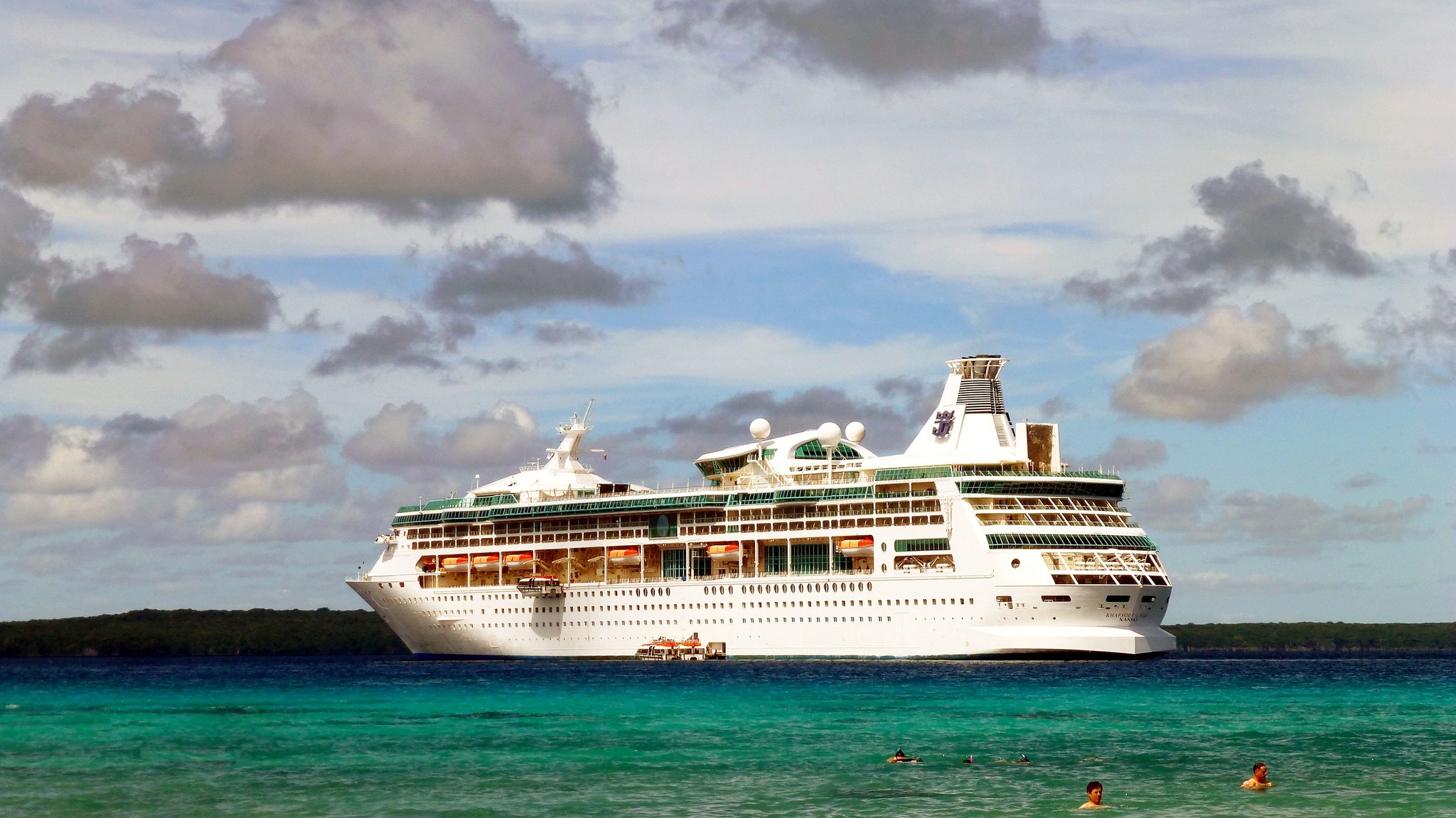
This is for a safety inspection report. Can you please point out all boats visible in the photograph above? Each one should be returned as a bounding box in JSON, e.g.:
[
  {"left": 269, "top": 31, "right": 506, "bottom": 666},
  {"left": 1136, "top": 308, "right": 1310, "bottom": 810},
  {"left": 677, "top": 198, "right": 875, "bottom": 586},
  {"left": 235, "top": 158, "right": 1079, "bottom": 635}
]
[
  {"left": 635, "top": 635, "right": 728, "bottom": 661},
  {"left": 516, "top": 574, "right": 562, "bottom": 598}
]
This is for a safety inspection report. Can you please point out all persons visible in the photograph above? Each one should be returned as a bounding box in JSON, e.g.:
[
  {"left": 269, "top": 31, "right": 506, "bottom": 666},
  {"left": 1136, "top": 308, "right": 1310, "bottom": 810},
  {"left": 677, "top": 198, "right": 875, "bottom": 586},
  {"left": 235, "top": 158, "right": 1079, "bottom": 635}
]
[
  {"left": 1080, "top": 781, "right": 1112, "bottom": 810},
  {"left": 639, "top": 635, "right": 726, "bottom": 659},
  {"left": 364, "top": 531, "right": 435, "bottom": 581},
  {"left": 988, "top": 754, "right": 1030, "bottom": 765},
  {"left": 528, "top": 577, "right": 575, "bottom": 588},
  {"left": 963, "top": 755, "right": 974, "bottom": 764},
  {"left": 1239, "top": 763, "right": 1275, "bottom": 791},
  {"left": 887, "top": 749, "right": 923, "bottom": 763}
]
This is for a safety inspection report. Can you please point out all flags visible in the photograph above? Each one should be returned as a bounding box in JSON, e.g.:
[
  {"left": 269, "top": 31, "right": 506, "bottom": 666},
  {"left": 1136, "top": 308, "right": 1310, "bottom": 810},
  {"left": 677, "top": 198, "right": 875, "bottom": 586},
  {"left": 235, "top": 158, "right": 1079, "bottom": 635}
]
[{"left": 603, "top": 453, "right": 607, "bottom": 461}]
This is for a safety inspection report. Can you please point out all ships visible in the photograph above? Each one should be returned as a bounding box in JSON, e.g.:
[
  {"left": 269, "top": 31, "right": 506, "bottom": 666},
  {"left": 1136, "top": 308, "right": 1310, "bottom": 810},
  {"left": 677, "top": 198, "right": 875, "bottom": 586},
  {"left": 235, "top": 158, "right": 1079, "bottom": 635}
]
[{"left": 347, "top": 353, "right": 1177, "bottom": 662}]
